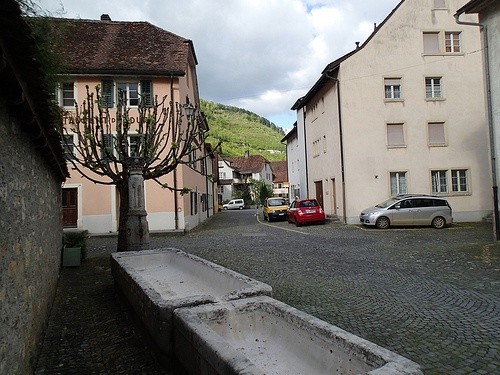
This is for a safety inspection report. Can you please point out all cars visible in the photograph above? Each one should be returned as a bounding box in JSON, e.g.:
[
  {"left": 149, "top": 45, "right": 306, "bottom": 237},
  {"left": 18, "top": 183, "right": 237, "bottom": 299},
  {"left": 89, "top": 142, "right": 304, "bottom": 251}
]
[{"left": 288, "top": 198, "right": 326, "bottom": 227}]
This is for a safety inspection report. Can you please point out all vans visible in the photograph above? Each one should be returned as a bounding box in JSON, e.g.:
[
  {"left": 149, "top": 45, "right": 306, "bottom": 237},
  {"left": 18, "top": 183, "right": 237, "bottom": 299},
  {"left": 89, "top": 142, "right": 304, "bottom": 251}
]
[{"left": 359, "top": 193, "right": 453, "bottom": 229}]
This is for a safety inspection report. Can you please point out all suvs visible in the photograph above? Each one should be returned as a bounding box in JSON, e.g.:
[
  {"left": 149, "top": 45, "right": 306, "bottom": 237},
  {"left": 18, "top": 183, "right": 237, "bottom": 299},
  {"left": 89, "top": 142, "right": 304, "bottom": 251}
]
[
  {"left": 223, "top": 198, "right": 245, "bottom": 210},
  {"left": 263, "top": 197, "right": 289, "bottom": 222}
]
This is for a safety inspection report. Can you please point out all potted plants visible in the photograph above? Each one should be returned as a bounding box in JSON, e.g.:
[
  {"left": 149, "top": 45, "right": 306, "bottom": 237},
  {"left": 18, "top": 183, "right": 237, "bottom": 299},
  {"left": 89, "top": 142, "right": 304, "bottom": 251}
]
[{"left": 62, "top": 232, "right": 89, "bottom": 267}]
